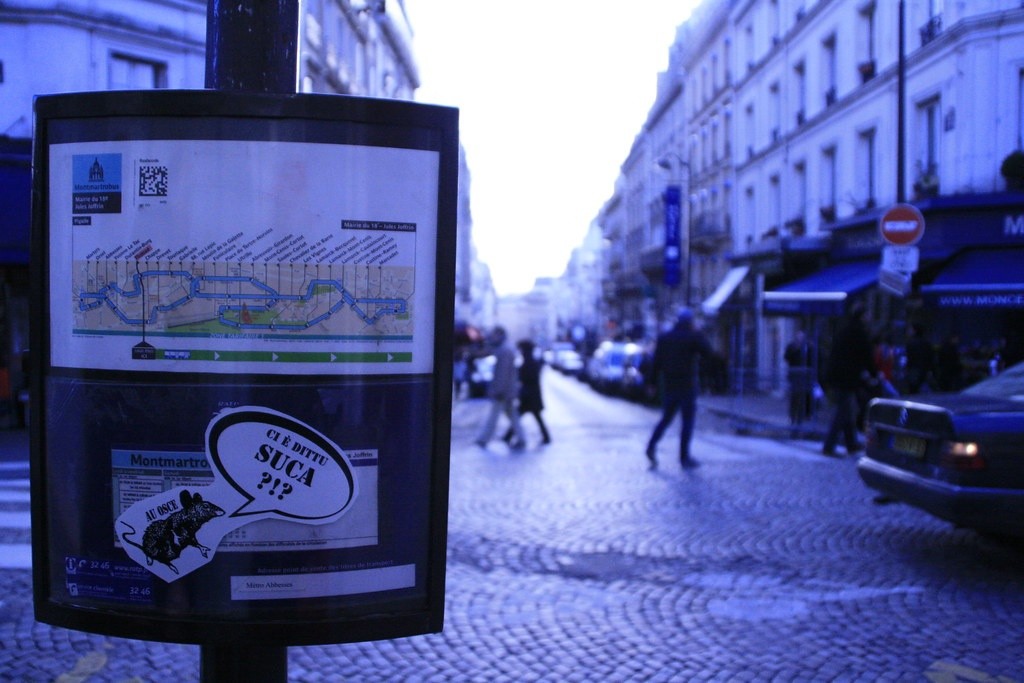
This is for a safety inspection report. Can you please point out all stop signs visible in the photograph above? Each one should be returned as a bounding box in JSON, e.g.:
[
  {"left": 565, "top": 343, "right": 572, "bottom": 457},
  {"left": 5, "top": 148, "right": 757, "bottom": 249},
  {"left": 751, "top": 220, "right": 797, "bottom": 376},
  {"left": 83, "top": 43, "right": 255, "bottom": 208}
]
[{"left": 881, "top": 204, "right": 926, "bottom": 246}]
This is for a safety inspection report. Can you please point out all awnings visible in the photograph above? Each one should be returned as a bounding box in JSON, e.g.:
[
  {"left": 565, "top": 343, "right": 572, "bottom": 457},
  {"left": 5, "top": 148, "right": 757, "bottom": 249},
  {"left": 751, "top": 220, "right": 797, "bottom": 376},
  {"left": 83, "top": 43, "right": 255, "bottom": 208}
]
[
  {"left": 919, "top": 248, "right": 1024, "bottom": 309},
  {"left": 702, "top": 263, "right": 755, "bottom": 318},
  {"left": 764, "top": 258, "right": 881, "bottom": 317}
]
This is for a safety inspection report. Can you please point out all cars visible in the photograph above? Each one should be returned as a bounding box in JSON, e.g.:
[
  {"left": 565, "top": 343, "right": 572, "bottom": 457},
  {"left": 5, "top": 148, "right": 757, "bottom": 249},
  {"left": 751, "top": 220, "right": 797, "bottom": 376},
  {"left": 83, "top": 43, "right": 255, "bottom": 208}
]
[
  {"left": 857, "top": 362, "right": 1024, "bottom": 545},
  {"left": 539, "top": 336, "right": 657, "bottom": 406}
]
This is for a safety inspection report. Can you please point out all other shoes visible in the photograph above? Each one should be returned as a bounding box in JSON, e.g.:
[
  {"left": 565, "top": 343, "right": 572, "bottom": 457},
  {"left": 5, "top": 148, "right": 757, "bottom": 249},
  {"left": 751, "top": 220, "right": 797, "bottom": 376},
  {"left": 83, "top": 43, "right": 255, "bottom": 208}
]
[
  {"left": 473, "top": 439, "right": 487, "bottom": 448},
  {"left": 681, "top": 459, "right": 699, "bottom": 469},
  {"left": 822, "top": 449, "right": 846, "bottom": 459},
  {"left": 848, "top": 443, "right": 865, "bottom": 454},
  {"left": 539, "top": 439, "right": 551, "bottom": 446},
  {"left": 513, "top": 441, "right": 526, "bottom": 451},
  {"left": 647, "top": 447, "right": 658, "bottom": 465},
  {"left": 498, "top": 436, "right": 510, "bottom": 445}
]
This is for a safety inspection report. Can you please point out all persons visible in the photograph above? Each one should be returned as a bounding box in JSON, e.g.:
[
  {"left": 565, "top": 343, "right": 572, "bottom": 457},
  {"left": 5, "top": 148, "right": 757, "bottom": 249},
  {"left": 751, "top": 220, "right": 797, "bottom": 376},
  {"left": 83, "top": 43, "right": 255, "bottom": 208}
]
[
  {"left": 500, "top": 339, "right": 552, "bottom": 446},
  {"left": 782, "top": 329, "right": 815, "bottom": 425},
  {"left": 902, "top": 323, "right": 963, "bottom": 392},
  {"left": 477, "top": 327, "right": 527, "bottom": 449},
  {"left": 641, "top": 307, "right": 719, "bottom": 468},
  {"left": 821, "top": 308, "right": 874, "bottom": 458}
]
